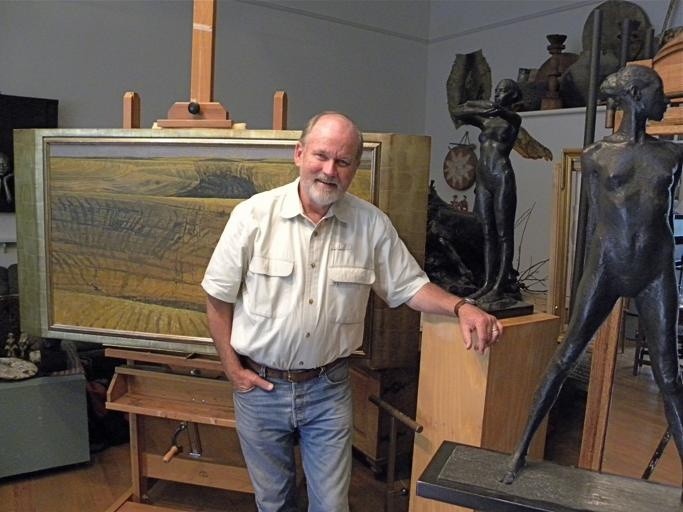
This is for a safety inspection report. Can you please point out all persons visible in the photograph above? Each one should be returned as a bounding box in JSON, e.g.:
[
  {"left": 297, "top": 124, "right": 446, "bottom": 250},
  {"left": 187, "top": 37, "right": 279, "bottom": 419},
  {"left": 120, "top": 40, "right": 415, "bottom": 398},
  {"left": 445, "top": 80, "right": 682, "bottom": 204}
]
[
  {"left": 201, "top": 111, "right": 503, "bottom": 512},
  {"left": 453, "top": 79, "right": 522, "bottom": 304},
  {"left": 499, "top": 64, "right": 683, "bottom": 484}
]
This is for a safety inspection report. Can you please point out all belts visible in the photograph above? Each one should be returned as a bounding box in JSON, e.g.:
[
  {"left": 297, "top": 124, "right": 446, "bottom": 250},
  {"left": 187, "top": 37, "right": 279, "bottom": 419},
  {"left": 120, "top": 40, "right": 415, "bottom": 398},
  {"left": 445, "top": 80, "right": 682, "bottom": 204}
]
[{"left": 243, "top": 356, "right": 343, "bottom": 385}]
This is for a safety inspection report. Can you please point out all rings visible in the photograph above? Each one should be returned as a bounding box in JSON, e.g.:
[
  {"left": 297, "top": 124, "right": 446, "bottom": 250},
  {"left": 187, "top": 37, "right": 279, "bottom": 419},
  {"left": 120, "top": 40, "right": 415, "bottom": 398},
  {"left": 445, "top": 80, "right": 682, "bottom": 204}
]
[{"left": 491, "top": 328, "right": 499, "bottom": 331}]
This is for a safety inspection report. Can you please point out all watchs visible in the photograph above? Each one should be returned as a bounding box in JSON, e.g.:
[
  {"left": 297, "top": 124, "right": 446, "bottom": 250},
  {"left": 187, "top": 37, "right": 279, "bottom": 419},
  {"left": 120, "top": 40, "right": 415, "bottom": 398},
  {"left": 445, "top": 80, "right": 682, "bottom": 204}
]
[{"left": 454, "top": 297, "right": 478, "bottom": 318}]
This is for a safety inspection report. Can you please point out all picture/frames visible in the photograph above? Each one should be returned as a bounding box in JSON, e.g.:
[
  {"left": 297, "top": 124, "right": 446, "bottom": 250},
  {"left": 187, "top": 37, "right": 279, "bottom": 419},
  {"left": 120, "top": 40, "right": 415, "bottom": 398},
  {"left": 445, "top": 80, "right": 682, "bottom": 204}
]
[
  {"left": 546, "top": 149, "right": 581, "bottom": 338},
  {"left": 33, "top": 131, "right": 387, "bottom": 371}
]
